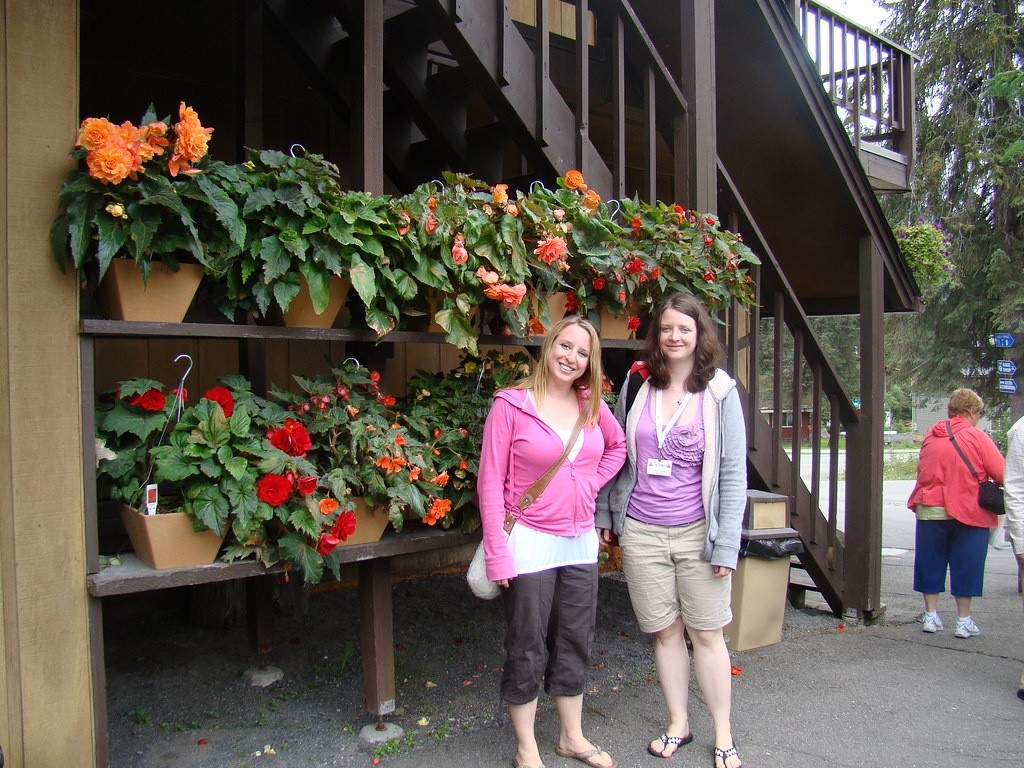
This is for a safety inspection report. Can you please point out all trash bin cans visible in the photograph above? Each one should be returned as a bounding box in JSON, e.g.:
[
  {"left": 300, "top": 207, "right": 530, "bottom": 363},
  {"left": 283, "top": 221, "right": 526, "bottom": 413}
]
[{"left": 725, "top": 489, "right": 803, "bottom": 649}]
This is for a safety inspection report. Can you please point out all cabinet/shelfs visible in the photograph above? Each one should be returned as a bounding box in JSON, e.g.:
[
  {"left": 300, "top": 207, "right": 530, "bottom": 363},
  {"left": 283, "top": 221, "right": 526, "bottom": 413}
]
[{"left": 80, "top": 319, "right": 648, "bottom": 716}]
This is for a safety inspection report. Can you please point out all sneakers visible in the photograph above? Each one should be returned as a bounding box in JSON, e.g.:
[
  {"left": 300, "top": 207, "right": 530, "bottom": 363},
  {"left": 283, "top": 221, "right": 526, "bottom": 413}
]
[
  {"left": 954, "top": 619, "right": 978, "bottom": 638},
  {"left": 923, "top": 613, "right": 943, "bottom": 632}
]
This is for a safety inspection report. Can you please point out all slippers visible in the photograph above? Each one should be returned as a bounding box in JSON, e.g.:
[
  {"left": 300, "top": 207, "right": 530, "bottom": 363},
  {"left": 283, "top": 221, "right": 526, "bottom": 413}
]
[
  {"left": 714, "top": 741, "right": 741, "bottom": 768},
  {"left": 513, "top": 758, "right": 545, "bottom": 768},
  {"left": 555, "top": 744, "right": 618, "bottom": 768},
  {"left": 649, "top": 732, "right": 693, "bottom": 757}
]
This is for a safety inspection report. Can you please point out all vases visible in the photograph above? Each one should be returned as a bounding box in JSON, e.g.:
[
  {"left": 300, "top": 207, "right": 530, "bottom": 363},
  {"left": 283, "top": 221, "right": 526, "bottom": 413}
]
[
  {"left": 97, "top": 256, "right": 205, "bottom": 323},
  {"left": 326, "top": 494, "right": 390, "bottom": 548},
  {"left": 586, "top": 296, "right": 641, "bottom": 340},
  {"left": 414, "top": 280, "right": 479, "bottom": 332},
  {"left": 524, "top": 289, "right": 569, "bottom": 335},
  {"left": 118, "top": 501, "right": 230, "bottom": 569},
  {"left": 272, "top": 271, "right": 354, "bottom": 327}
]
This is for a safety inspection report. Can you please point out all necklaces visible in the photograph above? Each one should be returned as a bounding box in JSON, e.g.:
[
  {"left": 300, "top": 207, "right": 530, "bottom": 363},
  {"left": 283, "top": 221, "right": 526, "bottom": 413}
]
[{"left": 669, "top": 384, "right": 685, "bottom": 405}]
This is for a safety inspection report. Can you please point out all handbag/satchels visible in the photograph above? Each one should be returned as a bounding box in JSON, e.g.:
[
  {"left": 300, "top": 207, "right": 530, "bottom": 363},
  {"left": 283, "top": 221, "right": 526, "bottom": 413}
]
[
  {"left": 979, "top": 481, "right": 1006, "bottom": 516},
  {"left": 468, "top": 525, "right": 510, "bottom": 600}
]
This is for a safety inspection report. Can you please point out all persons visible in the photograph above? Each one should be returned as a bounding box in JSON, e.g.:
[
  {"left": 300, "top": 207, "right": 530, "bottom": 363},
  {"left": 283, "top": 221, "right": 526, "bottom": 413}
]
[
  {"left": 907, "top": 388, "right": 1006, "bottom": 638},
  {"left": 594, "top": 292, "right": 747, "bottom": 768},
  {"left": 1003, "top": 415, "right": 1024, "bottom": 614},
  {"left": 478, "top": 316, "right": 627, "bottom": 768}
]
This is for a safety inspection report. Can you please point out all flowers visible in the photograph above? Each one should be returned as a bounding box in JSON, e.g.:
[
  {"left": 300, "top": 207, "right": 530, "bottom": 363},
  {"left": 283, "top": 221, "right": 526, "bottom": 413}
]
[
  {"left": 892, "top": 214, "right": 957, "bottom": 298},
  {"left": 44, "top": 101, "right": 762, "bottom": 583}
]
